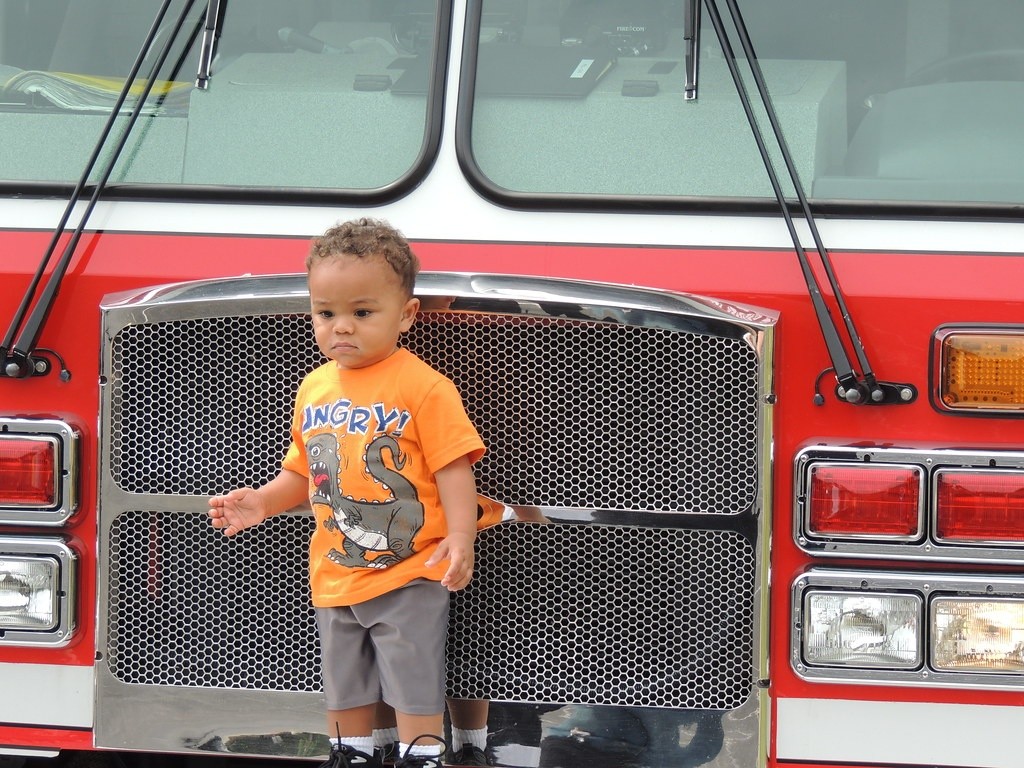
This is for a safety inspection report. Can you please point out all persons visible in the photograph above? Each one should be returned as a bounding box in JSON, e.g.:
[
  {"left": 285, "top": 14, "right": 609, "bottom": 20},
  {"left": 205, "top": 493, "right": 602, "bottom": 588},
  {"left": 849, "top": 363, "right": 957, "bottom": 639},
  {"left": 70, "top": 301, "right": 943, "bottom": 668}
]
[{"left": 208, "top": 218, "right": 487, "bottom": 768}]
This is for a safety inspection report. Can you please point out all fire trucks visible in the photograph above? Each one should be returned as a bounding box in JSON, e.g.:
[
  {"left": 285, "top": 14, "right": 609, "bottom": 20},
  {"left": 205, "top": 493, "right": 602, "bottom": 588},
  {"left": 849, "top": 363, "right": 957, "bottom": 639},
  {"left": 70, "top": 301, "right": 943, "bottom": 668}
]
[{"left": 0, "top": 0, "right": 1022, "bottom": 768}]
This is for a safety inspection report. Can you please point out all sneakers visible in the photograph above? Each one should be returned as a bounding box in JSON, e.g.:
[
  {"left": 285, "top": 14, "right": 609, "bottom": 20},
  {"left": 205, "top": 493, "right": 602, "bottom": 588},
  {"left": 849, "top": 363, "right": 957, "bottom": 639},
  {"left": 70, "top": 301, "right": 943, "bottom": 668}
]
[
  {"left": 449, "top": 745, "right": 488, "bottom": 766},
  {"left": 375, "top": 741, "right": 399, "bottom": 768},
  {"left": 321, "top": 721, "right": 375, "bottom": 768},
  {"left": 394, "top": 734, "right": 447, "bottom": 768}
]
